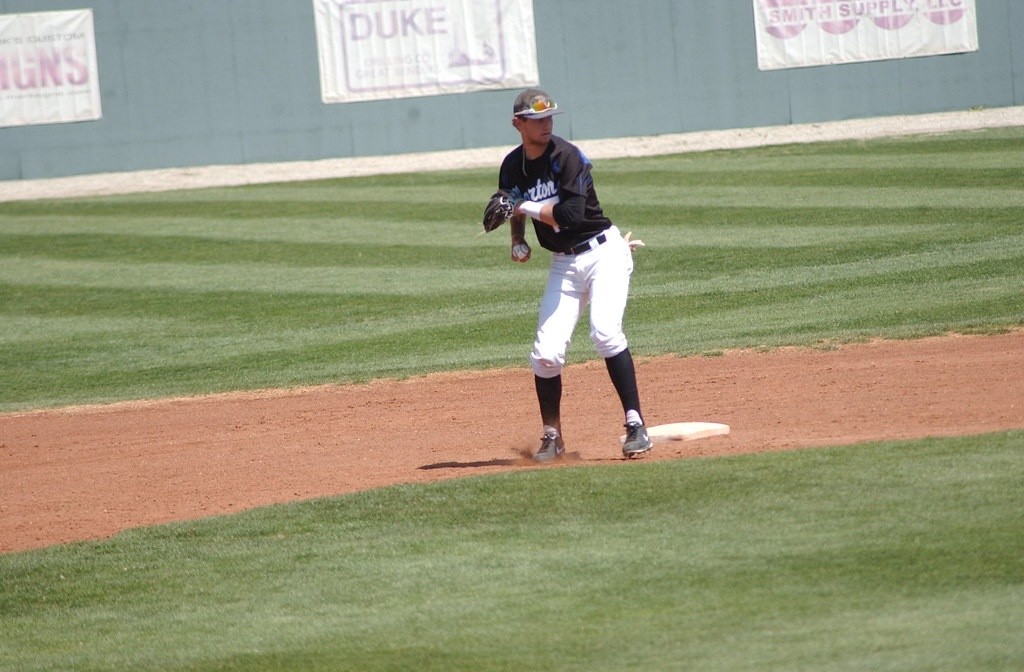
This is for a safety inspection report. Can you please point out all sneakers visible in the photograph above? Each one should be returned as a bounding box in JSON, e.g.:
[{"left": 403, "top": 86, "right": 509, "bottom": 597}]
[
  {"left": 624, "top": 423, "right": 652, "bottom": 457},
  {"left": 532, "top": 435, "right": 566, "bottom": 465}
]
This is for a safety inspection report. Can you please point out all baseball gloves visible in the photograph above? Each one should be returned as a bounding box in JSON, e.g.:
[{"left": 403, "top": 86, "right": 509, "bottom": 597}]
[{"left": 483, "top": 190, "right": 523, "bottom": 233}]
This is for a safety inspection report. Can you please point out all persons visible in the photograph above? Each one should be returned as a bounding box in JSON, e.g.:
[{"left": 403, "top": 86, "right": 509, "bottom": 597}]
[{"left": 497, "top": 88, "right": 651, "bottom": 461}]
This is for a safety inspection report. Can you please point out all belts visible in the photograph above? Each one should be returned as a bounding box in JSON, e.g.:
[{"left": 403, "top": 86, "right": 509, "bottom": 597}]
[{"left": 555, "top": 233, "right": 606, "bottom": 256}]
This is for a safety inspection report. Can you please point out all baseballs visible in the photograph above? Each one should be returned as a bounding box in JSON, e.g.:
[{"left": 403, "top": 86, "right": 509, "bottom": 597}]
[{"left": 513, "top": 244, "right": 528, "bottom": 261}]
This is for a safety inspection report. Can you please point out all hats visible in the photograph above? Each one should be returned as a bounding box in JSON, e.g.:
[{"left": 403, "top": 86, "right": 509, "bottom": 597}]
[{"left": 513, "top": 89, "right": 563, "bottom": 121}]
